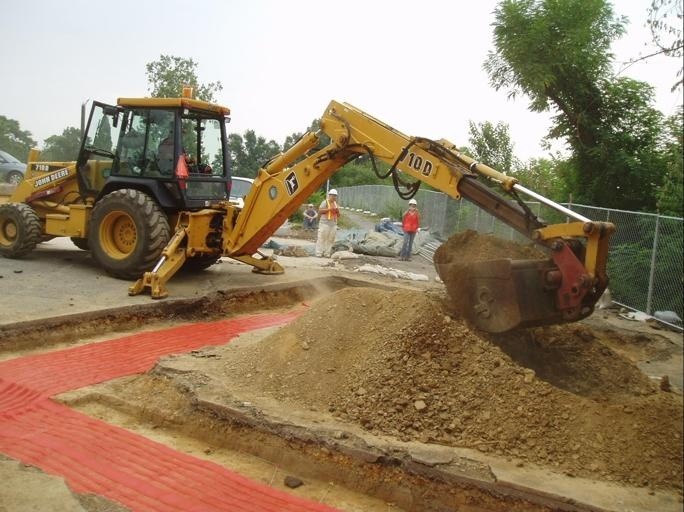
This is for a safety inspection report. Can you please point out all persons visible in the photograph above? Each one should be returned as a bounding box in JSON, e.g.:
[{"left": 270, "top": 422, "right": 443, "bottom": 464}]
[
  {"left": 315, "top": 189, "right": 340, "bottom": 258},
  {"left": 158, "top": 121, "right": 190, "bottom": 172},
  {"left": 398, "top": 198, "right": 419, "bottom": 261},
  {"left": 303, "top": 204, "right": 318, "bottom": 231}
]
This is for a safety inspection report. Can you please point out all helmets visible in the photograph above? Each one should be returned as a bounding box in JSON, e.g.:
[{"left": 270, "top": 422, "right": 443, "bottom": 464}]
[
  {"left": 328, "top": 189, "right": 338, "bottom": 196},
  {"left": 409, "top": 199, "right": 417, "bottom": 205}
]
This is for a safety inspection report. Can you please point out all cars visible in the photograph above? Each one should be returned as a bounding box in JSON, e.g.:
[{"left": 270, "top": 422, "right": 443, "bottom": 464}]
[
  {"left": 0, "top": 150, "right": 30, "bottom": 185},
  {"left": 205, "top": 175, "right": 255, "bottom": 206}
]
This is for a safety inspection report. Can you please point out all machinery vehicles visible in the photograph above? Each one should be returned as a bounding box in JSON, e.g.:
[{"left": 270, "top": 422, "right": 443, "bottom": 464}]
[{"left": 0, "top": 84, "right": 616, "bottom": 337}]
